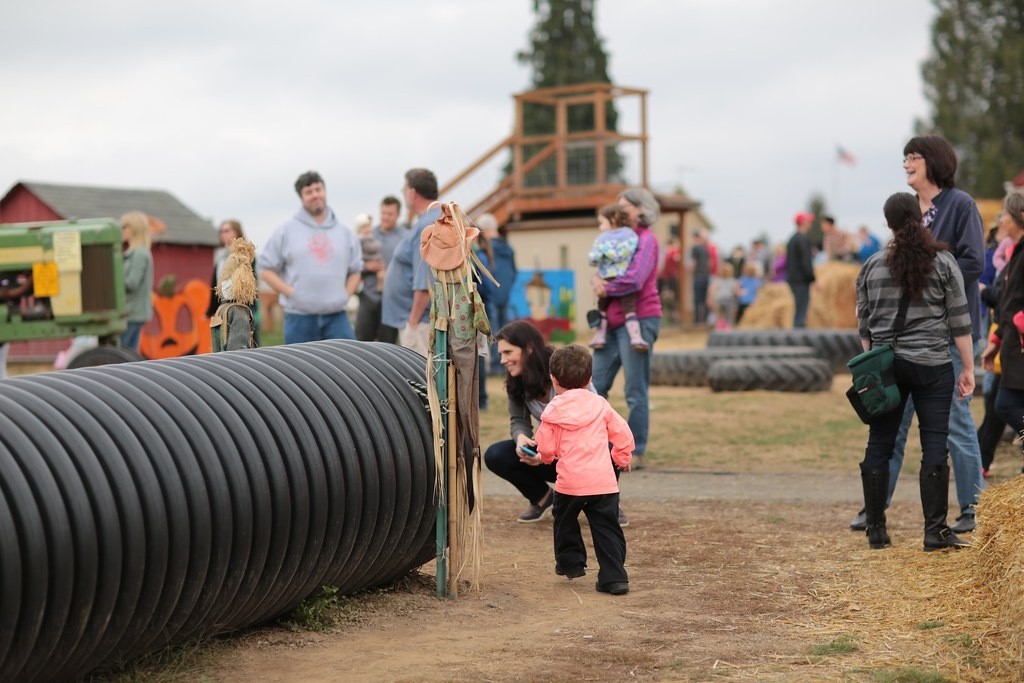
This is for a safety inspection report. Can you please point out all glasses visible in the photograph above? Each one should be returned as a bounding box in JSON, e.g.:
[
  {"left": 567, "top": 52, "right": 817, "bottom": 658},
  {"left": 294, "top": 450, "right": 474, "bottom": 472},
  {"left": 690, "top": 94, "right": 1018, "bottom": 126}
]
[{"left": 904, "top": 156, "right": 926, "bottom": 163}]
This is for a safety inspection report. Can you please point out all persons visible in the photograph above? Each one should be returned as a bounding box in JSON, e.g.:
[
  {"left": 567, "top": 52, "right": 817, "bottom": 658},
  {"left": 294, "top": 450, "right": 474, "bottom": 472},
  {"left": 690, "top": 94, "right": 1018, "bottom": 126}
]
[
  {"left": 852, "top": 135, "right": 986, "bottom": 532},
  {"left": 355, "top": 198, "right": 410, "bottom": 344},
  {"left": 257, "top": 172, "right": 363, "bottom": 340},
  {"left": 663, "top": 211, "right": 881, "bottom": 329},
  {"left": 588, "top": 185, "right": 662, "bottom": 469},
  {"left": 484, "top": 321, "right": 629, "bottom": 527},
  {"left": 856, "top": 192, "right": 975, "bottom": 551},
  {"left": 468, "top": 213, "right": 518, "bottom": 408},
  {"left": 978, "top": 192, "right": 1024, "bottom": 476},
  {"left": 536, "top": 345, "right": 634, "bottom": 594},
  {"left": 115, "top": 212, "right": 154, "bottom": 345},
  {"left": 206, "top": 219, "right": 258, "bottom": 316},
  {"left": 382, "top": 169, "right": 447, "bottom": 353}
]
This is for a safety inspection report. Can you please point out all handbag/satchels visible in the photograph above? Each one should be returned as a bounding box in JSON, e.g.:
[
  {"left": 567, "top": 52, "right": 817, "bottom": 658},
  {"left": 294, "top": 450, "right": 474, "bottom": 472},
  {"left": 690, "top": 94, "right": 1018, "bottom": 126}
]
[{"left": 846, "top": 345, "right": 900, "bottom": 423}]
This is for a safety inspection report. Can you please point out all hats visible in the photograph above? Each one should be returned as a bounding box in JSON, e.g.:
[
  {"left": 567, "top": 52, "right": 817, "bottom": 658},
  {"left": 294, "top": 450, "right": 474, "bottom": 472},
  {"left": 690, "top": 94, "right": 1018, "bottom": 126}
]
[{"left": 796, "top": 213, "right": 815, "bottom": 224}]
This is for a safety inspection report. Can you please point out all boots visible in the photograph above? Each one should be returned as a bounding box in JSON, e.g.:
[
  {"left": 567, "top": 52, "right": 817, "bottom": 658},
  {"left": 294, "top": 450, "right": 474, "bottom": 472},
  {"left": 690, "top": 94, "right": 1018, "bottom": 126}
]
[
  {"left": 920, "top": 466, "right": 971, "bottom": 551},
  {"left": 625, "top": 317, "right": 648, "bottom": 352},
  {"left": 589, "top": 317, "right": 608, "bottom": 349},
  {"left": 859, "top": 462, "right": 890, "bottom": 547}
]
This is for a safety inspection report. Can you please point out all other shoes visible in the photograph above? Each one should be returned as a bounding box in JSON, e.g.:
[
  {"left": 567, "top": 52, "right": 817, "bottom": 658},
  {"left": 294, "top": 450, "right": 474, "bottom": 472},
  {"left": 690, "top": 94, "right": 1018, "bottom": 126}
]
[
  {"left": 630, "top": 454, "right": 646, "bottom": 470},
  {"left": 518, "top": 489, "right": 554, "bottom": 522},
  {"left": 555, "top": 563, "right": 586, "bottom": 579},
  {"left": 618, "top": 504, "right": 629, "bottom": 527},
  {"left": 851, "top": 504, "right": 867, "bottom": 530},
  {"left": 595, "top": 581, "right": 628, "bottom": 594},
  {"left": 951, "top": 513, "right": 977, "bottom": 533}
]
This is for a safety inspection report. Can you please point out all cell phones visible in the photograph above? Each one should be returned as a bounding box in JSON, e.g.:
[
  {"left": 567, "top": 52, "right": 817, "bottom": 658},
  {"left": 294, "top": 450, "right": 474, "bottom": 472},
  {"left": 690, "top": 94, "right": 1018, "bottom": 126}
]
[{"left": 520, "top": 443, "right": 538, "bottom": 456}]
